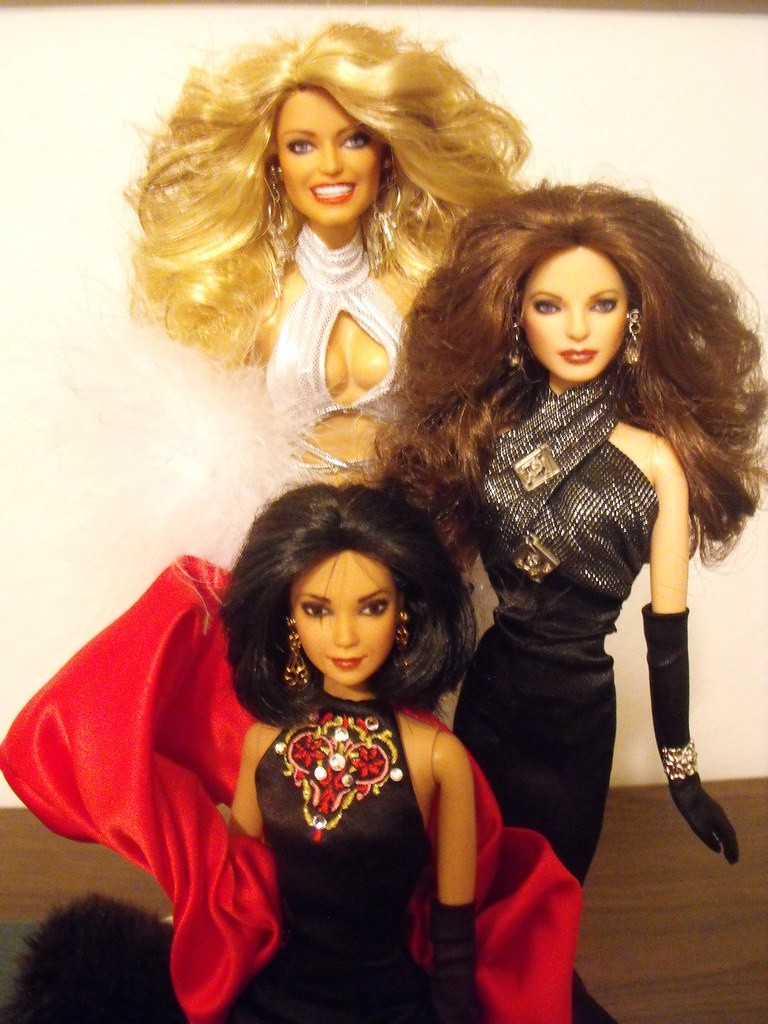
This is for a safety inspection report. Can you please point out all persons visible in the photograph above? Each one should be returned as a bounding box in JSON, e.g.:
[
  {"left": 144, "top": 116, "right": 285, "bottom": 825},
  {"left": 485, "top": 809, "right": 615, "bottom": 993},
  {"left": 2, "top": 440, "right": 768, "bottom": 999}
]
[
  {"left": 218, "top": 482, "right": 478, "bottom": 1024},
  {"left": 367, "top": 188, "right": 768, "bottom": 1024},
  {"left": 132, "top": 25, "right": 530, "bottom": 478}
]
